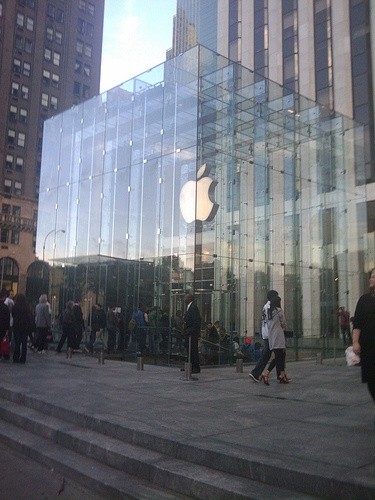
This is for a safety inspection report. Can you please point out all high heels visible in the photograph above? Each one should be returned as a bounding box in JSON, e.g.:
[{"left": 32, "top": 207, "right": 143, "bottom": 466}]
[
  {"left": 260, "top": 370, "right": 270, "bottom": 385},
  {"left": 279, "top": 373, "right": 290, "bottom": 384}
]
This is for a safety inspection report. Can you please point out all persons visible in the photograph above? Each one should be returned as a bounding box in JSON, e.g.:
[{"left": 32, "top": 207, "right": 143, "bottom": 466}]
[
  {"left": 352, "top": 268, "right": 375, "bottom": 404},
  {"left": 86, "top": 303, "right": 260, "bottom": 365},
  {"left": 0, "top": 291, "right": 55, "bottom": 360},
  {"left": 56, "top": 300, "right": 84, "bottom": 353},
  {"left": 181, "top": 293, "right": 202, "bottom": 373},
  {"left": 35, "top": 294, "right": 52, "bottom": 354},
  {"left": 261, "top": 296, "right": 289, "bottom": 386},
  {"left": 335, "top": 306, "right": 353, "bottom": 347},
  {"left": 12, "top": 294, "right": 32, "bottom": 364},
  {"left": 249, "top": 290, "right": 281, "bottom": 383}
]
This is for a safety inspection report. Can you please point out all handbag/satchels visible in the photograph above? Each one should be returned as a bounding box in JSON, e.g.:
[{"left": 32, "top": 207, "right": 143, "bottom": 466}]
[{"left": 0, "top": 330, "right": 10, "bottom": 356}]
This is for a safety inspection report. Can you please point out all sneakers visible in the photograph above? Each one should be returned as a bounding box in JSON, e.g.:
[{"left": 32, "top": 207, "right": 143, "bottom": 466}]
[
  {"left": 276, "top": 376, "right": 293, "bottom": 382},
  {"left": 248, "top": 370, "right": 260, "bottom": 383}
]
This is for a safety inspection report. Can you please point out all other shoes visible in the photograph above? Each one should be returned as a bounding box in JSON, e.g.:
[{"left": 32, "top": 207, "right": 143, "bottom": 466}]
[
  {"left": 181, "top": 368, "right": 200, "bottom": 373},
  {"left": 14, "top": 357, "right": 25, "bottom": 363}
]
